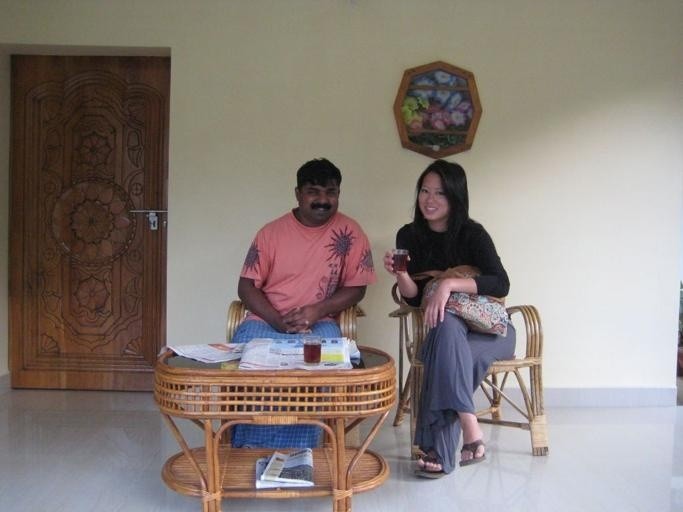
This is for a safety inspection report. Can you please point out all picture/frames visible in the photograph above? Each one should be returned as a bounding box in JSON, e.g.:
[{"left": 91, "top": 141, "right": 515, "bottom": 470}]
[{"left": 394, "top": 61, "right": 483, "bottom": 160}]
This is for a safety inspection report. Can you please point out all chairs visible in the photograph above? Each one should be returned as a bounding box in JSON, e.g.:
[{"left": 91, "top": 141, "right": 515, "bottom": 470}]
[
  {"left": 222, "top": 301, "right": 357, "bottom": 445},
  {"left": 390, "top": 283, "right": 549, "bottom": 460}
]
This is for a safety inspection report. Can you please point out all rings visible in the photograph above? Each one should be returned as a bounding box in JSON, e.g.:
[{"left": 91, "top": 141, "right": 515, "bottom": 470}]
[{"left": 304, "top": 320, "right": 309, "bottom": 324}]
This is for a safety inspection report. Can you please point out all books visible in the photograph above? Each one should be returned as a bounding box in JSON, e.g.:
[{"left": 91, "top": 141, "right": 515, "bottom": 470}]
[{"left": 253, "top": 446, "right": 315, "bottom": 492}]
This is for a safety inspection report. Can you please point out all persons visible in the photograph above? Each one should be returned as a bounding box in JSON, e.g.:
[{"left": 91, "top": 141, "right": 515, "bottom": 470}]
[
  {"left": 382, "top": 161, "right": 517, "bottom": 481},
  {"left": 226, "top": 157, "right": 378, "bottom": 450}
]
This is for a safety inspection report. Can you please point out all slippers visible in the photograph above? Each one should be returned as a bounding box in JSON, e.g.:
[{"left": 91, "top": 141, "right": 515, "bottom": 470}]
[
  {"left": 459, "top": 439, "right": 486, "bottom": 468},
  {"left": 413, "top": 454, "right": 449, "bottom": 480}
]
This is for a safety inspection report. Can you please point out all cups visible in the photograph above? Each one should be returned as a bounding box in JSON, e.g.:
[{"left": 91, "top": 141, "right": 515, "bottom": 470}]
[
  {"left": 393, "top": 247, "right": 409, "bottom": 272},
  {"left": 303, "top": 334, "right": 323, "bottom": 364}
]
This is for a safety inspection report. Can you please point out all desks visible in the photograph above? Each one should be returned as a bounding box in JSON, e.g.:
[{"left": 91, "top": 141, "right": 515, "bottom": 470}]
[{"left": 153, "top": 346, "right": 396, "bottom": 512}]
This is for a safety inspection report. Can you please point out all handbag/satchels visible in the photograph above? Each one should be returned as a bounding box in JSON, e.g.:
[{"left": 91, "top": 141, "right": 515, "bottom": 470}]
[{"left": 390, "top": 265, "right": 509, "bottom": 340}]
